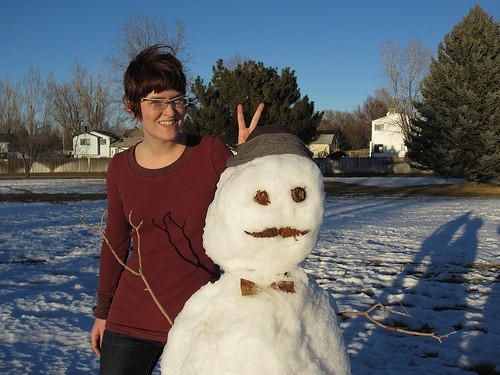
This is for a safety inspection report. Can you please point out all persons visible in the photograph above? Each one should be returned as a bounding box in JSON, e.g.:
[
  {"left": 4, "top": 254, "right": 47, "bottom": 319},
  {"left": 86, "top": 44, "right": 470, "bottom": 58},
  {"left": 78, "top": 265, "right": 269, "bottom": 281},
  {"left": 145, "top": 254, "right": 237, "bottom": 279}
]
[{"left": 88, "top": 42, "right": 266, "bottom": 374}]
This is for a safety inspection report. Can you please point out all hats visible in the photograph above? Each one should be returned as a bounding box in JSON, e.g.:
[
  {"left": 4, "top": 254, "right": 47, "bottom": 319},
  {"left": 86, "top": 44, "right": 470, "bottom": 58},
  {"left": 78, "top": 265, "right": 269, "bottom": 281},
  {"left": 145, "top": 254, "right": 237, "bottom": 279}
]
[{"left": 227, "top": 125, "right": 314, "bottom": 166}]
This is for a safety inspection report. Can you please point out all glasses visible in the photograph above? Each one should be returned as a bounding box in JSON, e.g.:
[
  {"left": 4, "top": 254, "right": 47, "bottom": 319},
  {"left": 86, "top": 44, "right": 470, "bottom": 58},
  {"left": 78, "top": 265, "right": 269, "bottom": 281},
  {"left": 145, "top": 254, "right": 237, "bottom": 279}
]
[{"left": 140, "top": 94, "right": 190, "bottom": 110}]
